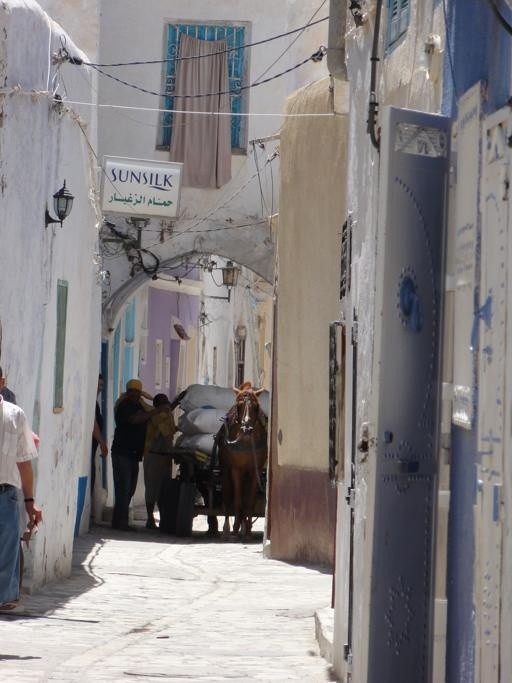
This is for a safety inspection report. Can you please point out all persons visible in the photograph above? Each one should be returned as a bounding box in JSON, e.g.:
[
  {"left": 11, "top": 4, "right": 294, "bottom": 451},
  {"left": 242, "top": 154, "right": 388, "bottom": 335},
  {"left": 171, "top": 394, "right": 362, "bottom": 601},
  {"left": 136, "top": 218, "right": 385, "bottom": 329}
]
[
  {"left": 111, "top": 379, "right": 171, "bottom": 530},
  {"left": 91, "top": 373, "right": 109, "bottom": 493},
  {"left": 0, "top": 366, "right": 45, "bottom": 609},
  {"left": 0, "top": 385, "right": 40, "bottom": 600},
  {"left": 145, "top": 387, "right": 186, "bottom": 530}
]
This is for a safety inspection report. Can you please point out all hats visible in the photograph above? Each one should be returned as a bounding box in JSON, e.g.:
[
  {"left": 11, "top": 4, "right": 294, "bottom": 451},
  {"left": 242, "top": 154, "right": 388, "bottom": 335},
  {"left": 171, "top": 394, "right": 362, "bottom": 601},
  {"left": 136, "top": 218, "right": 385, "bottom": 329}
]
[{"left": 125, "top": 379, "right": 143, "bottom": 392}]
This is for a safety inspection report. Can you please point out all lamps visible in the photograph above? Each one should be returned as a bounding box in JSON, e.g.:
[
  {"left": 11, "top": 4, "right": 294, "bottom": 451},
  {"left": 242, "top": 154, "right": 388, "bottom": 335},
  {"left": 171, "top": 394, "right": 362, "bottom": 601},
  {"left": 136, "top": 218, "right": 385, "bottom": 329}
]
[
  {"left": 41, "top": 176, "right": 73, "bottom": 230},
  {"left": 215, "top": 258, "right": 242, "bottom": 302}
]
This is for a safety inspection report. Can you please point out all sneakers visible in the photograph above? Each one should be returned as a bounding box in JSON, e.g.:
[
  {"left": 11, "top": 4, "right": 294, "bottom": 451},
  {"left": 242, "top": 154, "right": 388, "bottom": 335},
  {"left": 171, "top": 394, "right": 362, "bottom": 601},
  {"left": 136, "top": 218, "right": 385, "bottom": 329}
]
[{"left": 110, "top": 518, "right": 160, "bottom": 532}]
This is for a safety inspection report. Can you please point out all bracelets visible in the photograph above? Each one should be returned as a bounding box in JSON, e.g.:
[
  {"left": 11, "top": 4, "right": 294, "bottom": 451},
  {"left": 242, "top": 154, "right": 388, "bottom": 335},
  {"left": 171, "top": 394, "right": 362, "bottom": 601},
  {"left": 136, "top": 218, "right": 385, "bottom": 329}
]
[{"left": 23, "top": 496, "right": 35, "bottom": 502}]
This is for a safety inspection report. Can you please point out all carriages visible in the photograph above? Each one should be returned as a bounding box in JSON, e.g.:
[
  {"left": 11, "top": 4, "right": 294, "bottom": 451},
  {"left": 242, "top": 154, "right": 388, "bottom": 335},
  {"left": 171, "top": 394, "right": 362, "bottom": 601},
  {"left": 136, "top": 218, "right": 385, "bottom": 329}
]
[{"left": 160, "top": 380, "right": 270, "bottom": 538}]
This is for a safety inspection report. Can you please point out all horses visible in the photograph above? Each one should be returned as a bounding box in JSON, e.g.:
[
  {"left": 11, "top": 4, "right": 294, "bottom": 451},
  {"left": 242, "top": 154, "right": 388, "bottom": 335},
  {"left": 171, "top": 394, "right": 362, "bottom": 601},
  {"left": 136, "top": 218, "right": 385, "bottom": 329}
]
[{"left": 223, "top": 387, "right": 267, "bottom": 544}]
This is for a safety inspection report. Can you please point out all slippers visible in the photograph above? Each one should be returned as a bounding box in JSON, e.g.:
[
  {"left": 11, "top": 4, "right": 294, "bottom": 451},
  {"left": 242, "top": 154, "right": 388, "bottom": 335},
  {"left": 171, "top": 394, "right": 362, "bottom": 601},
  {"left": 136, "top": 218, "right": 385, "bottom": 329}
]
[{"left": 0, "top": 602, "right": 25, "bottom": 615}]
